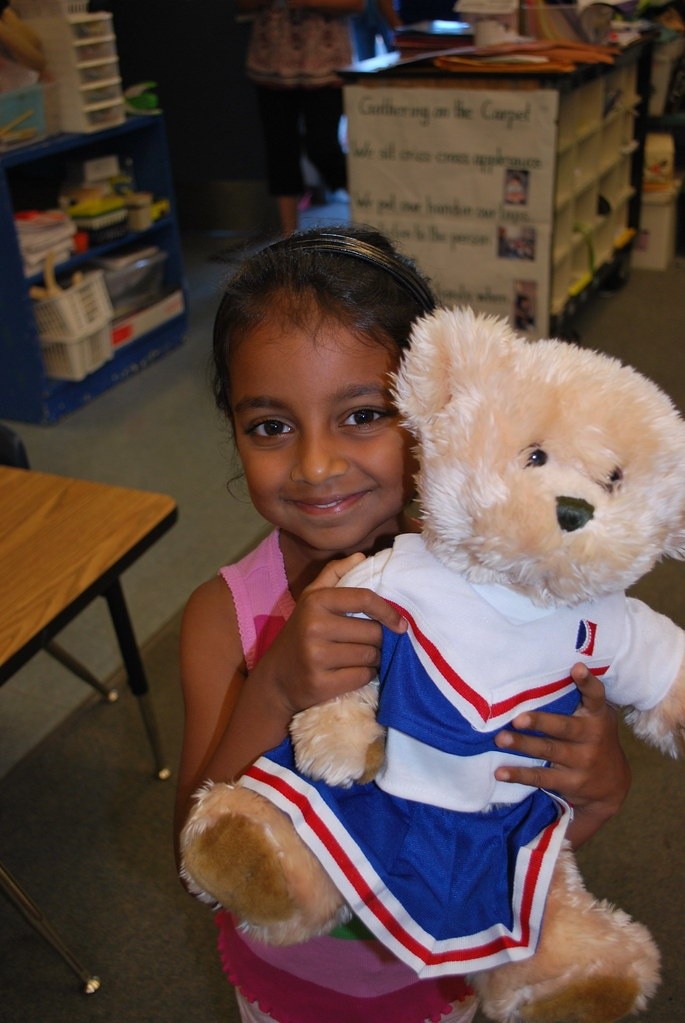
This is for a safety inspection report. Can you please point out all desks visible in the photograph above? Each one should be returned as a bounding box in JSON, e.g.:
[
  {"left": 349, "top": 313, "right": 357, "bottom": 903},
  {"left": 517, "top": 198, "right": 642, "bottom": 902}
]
[{"left": 0, "top": 466, "right": 178, "bottom": 992}]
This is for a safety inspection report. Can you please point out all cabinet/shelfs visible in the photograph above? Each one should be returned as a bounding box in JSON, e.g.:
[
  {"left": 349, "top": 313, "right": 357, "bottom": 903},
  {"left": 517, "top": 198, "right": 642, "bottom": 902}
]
[
  {"left": 334, "top": 30, "right": 655, "bottom": 340},
  {"left": 0, "top": 112, "right": 191, "bottom": 426}
]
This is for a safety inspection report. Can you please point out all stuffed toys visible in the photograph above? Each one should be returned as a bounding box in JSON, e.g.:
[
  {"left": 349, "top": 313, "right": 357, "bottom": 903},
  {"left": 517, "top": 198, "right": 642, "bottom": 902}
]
[{"left": 178, "top": 306, "right": 685, "bottom": 1022}]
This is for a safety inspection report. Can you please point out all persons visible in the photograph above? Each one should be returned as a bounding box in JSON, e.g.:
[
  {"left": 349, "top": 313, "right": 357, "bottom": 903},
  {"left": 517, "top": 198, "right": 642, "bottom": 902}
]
[
  {"left": 236, "top": 1, "right": 363, "bottom": 235},
  {"left": 174, "top": 224, "right": 636, "bottom": 1022}
]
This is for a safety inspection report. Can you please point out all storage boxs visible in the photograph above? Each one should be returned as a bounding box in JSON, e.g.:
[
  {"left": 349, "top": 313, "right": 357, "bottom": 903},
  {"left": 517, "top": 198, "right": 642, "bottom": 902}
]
[{"left": 628, "top": 178, "right": 681, "bottom": 269}]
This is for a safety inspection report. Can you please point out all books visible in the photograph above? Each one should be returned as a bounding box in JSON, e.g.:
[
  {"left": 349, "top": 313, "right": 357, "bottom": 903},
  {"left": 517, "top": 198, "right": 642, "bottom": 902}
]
[{"left": 8, "top": 153, "right": 173, "bottom": 278}]
